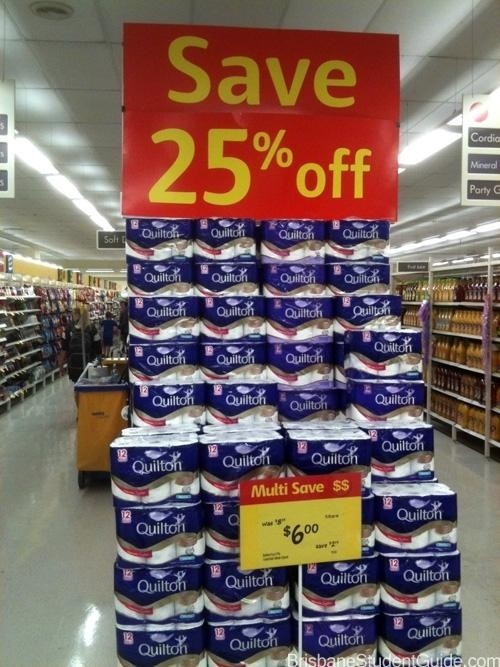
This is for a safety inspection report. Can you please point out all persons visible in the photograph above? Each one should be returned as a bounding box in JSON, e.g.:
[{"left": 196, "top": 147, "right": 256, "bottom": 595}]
[
  {"left": 118, "top": 301, "right": 129, "bottom": 353},
  {"left": 59, "top": 306, "right": 102, "bottom": 420},
  {"left": 101, "top": 311, "right": 119, "bottom": 357}
]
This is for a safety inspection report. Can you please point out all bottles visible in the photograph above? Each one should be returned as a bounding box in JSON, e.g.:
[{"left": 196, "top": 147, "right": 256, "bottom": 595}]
[{"left": 392, "top": 274, "right": 500, "bottom": 441}]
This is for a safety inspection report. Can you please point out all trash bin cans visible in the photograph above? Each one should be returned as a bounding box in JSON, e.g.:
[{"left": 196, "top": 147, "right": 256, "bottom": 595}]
[{"left": 72, "top": 363, "right": 128, "bottom": 490}]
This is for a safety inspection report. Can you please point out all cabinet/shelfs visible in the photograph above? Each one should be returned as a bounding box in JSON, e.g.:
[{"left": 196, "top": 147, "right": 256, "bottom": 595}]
[
  {"left": 389, "top": 247, "right": 500, "bottom": 458},
  {"left": 0, "top": 271, "right": 123, "bottom": 411}
]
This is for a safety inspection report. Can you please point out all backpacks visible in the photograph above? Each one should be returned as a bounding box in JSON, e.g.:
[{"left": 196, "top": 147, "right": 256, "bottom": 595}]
[{"left": 68, "top": 328, "right": 93, "bottom": 377}]
